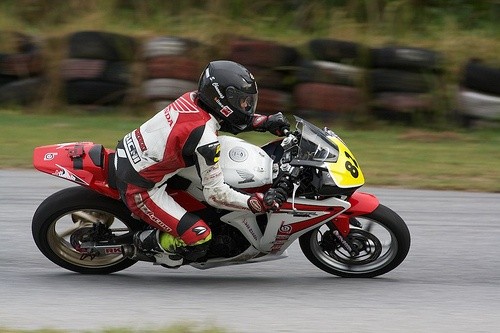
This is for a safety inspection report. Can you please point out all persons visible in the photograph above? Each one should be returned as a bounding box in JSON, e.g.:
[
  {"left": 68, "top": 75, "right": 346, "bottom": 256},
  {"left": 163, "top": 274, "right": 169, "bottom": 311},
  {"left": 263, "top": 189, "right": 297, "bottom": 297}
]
[{"left": 113, "top": 60, "right": 291, "bottom": 269}]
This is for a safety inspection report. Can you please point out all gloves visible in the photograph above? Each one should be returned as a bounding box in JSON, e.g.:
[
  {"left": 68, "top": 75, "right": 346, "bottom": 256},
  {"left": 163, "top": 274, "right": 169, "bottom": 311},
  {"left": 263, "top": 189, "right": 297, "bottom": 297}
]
[
  {"left": 248, "top": 188, "right": 288, "bottom": 214},
  {"left": 252, "top": 112, "right": 291, "bottom": 137}
]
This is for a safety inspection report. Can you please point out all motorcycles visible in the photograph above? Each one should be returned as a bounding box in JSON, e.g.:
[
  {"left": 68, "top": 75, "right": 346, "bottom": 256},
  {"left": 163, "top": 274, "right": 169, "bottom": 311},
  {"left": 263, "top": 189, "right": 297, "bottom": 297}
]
[{"left": 30, "top": 115, "right": 411, "bottom": 278}]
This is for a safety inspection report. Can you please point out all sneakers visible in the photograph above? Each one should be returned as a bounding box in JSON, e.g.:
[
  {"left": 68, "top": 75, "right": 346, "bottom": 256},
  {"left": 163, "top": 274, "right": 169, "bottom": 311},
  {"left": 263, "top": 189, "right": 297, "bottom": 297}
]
[{"left": 134, "top": 229, "right": 183, "bottom": 269}]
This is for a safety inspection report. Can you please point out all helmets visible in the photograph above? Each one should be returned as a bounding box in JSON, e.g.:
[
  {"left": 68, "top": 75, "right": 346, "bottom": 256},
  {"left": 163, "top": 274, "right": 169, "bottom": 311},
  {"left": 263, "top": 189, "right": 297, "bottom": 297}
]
[{"left": 198, "top": 61, "right": 258, "bottom": 134}]
